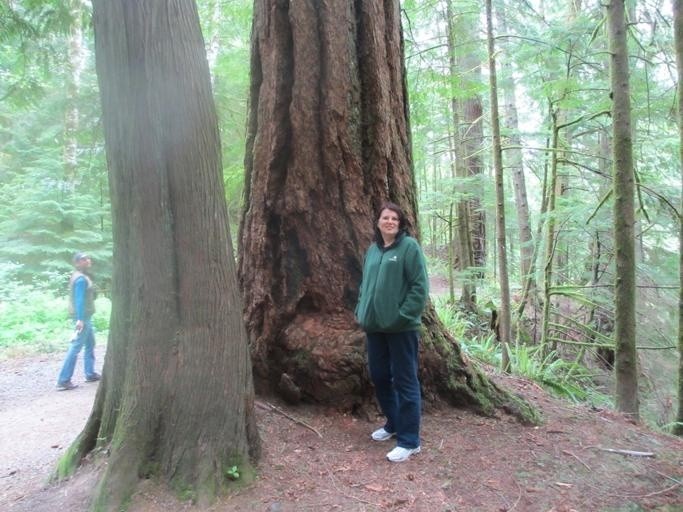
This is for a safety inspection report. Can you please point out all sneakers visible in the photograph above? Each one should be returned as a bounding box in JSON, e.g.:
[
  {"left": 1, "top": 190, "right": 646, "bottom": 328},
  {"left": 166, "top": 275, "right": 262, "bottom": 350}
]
[
  {"left": 370, "top": 423, "right": 397, "bottom": 442},
  {"left": 83, "top": 371, "right": 102, "bottom": 382},
  {"left": 54, "top": 379, "right": 79, "bottom": 392},
  {"left": 384, "top": 441, "right": 423, "bottom": 462}
]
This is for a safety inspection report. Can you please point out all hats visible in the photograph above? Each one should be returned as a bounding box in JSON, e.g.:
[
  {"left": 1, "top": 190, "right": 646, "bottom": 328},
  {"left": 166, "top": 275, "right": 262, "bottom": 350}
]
[{"left": 71, "top": 252, "right": 90, "bottom": 263}]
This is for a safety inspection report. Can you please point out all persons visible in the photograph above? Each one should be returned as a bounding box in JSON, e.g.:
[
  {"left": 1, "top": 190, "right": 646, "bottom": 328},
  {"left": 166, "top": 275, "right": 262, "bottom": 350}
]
[
  {"left": 54, "top": 250, "right": 103, "bottom": 391},
  {"left": 353, "top": 203, "right": 429, "bottom": 465}
]
